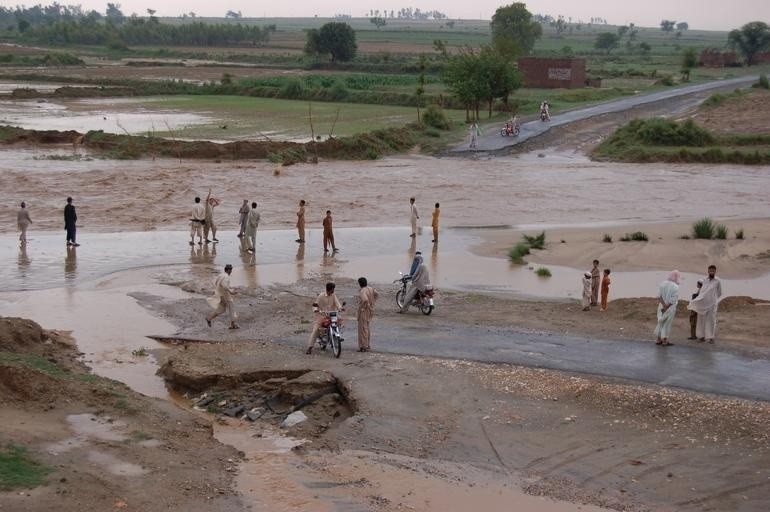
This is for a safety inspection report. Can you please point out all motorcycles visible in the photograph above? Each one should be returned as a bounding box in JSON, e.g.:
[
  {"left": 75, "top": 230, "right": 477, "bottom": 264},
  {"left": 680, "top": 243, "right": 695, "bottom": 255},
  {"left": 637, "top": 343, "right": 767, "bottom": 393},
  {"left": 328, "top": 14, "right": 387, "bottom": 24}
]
[
  {"left": 541, "top": 109, "right": 548, "bottom": 121},
  {"left": 391, "top": 271, "right": 434, "bottom": 315},
  {"left": 500, "top": 121, "right": 520, "bottom": 136},
  {"left": 311, "top": 301, "right": 349, "bottom": 358}
]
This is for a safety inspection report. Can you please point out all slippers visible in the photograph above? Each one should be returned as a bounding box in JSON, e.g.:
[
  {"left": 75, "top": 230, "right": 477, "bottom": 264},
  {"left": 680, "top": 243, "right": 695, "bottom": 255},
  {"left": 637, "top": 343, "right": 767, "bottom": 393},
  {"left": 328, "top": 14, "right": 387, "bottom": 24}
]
[
  {"left": 205, "top": 318, "right": 212, "bottom": 326},
  {"left": 228, "top": 326, "right": 240, "bottom": 329}
]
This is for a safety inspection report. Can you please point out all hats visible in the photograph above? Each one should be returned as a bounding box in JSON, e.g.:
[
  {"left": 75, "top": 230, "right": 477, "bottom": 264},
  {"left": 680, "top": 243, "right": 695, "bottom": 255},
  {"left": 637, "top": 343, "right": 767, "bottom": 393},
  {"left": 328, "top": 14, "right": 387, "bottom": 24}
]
[
  {"left": 225, "top": 264, "right": 233, "bottom": 269},
  {"left": 585, "top": 271, "right": 592, "bottom": 277}
]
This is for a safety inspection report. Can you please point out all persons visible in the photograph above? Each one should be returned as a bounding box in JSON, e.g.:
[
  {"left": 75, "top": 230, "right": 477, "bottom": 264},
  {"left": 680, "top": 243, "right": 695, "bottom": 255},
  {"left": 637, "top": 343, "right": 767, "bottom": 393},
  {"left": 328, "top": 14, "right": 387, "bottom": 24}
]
[
  {"left": 651, "top": 269, "right": 681, "bottom": 347},
  {"left": 304, "top": 283, "right": 346, "bottom": 355},
  {"left": 356, "top": 275, "right": 380, "bottom": 353},
  {"left": 295, "top": 198, "right": 307, "bottom": 244},
  {"left": 466, "top": 120, "right": 478, "bottom": 150},
  {"left": 599, "top": 268, "right": 611, "bottom": 312},
  {"left": 203, "top": 263, "right": 241, "bottom": 330},
  {"left": 431, "top": 202, "right": 440, "bottom": 243},
  {"left": 203, "top": 187, "right": 220, "bottom": 243},
  {"left": 15, "top": 201, "right": 33, "bottom": 246},
  {"left": 322, "top": 210, "right": 340, "bottom": 252},
  {"left": 408, "top": 196, "right": 420, "bottom": 237},
  {"left": 507, "top": 113, "right": 516, "bottom": 138},
  {"left": 236, "top": 198, "right": 250, "bottom": 238},
  {"left": 687, "top": 292, "right": 698, "bottom": 340},
  {"left": 243, "top": 201, "right": 262, "bottom": 253},
  {"left": 63, "top": 196, "right": 80, "bottom": 247},
  {"left": 537, "top": 100, "right": 551, "bottom": 122},
  {"left": 188, "top": 196, "right": 206, "bottom": 246},
  {"left": 696, "top": 279, "right": 703, "bottom": 295},
  {"left": 579, "top": 271, "right": 593, "bottom": 312},
  {"left": 395, "top": 255, "right": 431, "bottom": 315},
  {"left": 409, "top": 251, "right": 421, "bottom": 276},
  {"left": 696, "top": 264, "right": 722, "bottom": 345},
  {"left": 590, "top": 258, "right": 601, "bottom": 307}
]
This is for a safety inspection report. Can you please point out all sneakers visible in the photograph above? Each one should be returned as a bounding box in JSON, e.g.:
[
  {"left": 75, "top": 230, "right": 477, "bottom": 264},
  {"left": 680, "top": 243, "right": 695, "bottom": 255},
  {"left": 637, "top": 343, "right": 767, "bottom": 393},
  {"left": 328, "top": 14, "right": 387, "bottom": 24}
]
[
  {"left": 581, "top": 302, "right": 609, "bottom": 312},
  {"left": 400, "top": 308, "right": 408, "bottom": 313},
  {"left": 237, "top": 232, "right": 246, "bottom": 237},
  {"left": 189, "top": 238, "right": 219, "bottom": 245},
  {"left": 324, "top": 247, "right": 339, "bottom": 252},
  {"left": 248, "top": 248, "right": 255, "bottom": 251},
  {"left": 296, "top": 239, "right": 305, "bottom": 243},
  {"left": 305, "top": 349, "right": 312, "bottom": 355},
  {"left": 409, "top": 233, "right": 439, "bottom": 243},
  {"left": 687, "top": 336, "right": 714, "bottom": 344},
  {"left": 358, "top": 346, "right": 371, "bottom": 352},
  {"left": 655, "top": 340, "right": 673, "bottom": 347}
]
